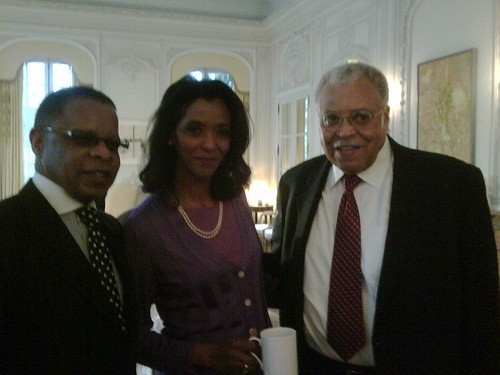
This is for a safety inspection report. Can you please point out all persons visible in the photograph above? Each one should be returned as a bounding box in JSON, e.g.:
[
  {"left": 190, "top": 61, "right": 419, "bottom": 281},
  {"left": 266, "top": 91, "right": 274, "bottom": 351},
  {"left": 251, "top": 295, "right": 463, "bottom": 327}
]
[
  {"left": 113, "top": 75, "right": 276, "bottom": 375},
  {"left": 0, "top": 87, "right": 136, "bottom": 375},
  {"left": 272, "top": 61, "right": 500, "bottom": 375}
]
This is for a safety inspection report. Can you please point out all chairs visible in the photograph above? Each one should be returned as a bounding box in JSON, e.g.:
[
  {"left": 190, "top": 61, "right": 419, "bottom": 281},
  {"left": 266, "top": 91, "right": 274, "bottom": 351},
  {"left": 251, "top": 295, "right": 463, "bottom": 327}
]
[{"left": 248, "top": 206, "right": 274, "bottom": 254}]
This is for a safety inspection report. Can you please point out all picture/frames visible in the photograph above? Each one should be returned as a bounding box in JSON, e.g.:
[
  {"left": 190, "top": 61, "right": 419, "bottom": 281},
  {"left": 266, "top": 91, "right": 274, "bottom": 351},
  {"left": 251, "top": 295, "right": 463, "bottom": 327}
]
[{"left": 416, "top": 48, "right": 476, "bottom": 168}]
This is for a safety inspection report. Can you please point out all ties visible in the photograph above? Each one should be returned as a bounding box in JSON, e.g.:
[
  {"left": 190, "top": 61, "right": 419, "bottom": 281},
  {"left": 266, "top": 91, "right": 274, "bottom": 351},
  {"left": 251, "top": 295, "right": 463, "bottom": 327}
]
[
  {"left": 74, "top": 206, "right": 128, "bottom": 340},
  {"left": 326, "top": 174, "right": 366, "bottom": 361}
]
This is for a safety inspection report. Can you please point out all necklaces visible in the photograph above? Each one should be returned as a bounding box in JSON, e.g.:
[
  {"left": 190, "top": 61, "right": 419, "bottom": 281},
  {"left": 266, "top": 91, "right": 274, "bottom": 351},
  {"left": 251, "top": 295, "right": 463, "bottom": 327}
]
[{"left": 168, "top": 190, "right": 223, "bottom": 240}]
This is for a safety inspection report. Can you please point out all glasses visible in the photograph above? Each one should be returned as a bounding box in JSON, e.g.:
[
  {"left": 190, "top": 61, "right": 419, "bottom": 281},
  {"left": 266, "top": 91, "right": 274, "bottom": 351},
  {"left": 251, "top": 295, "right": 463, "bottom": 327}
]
[
  {"left": 319, "top": 107, "right": 383, "bottom": 128},
  {"left": 44, "top": 127, "right": 129, "bottom": 155}
]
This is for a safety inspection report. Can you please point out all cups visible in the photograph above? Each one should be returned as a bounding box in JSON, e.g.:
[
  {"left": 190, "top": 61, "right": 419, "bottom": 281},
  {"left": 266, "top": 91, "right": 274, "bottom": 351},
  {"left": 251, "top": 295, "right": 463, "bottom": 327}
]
[{"left": 249, "top": 327, "right": 299, "bottom": 375}]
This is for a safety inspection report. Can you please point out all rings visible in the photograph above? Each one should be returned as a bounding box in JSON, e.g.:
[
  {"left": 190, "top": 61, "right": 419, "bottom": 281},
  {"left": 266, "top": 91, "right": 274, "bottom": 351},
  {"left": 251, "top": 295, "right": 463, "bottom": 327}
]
[{"left": 240, "top": 363, "right": 249, "bottom": 374}]
